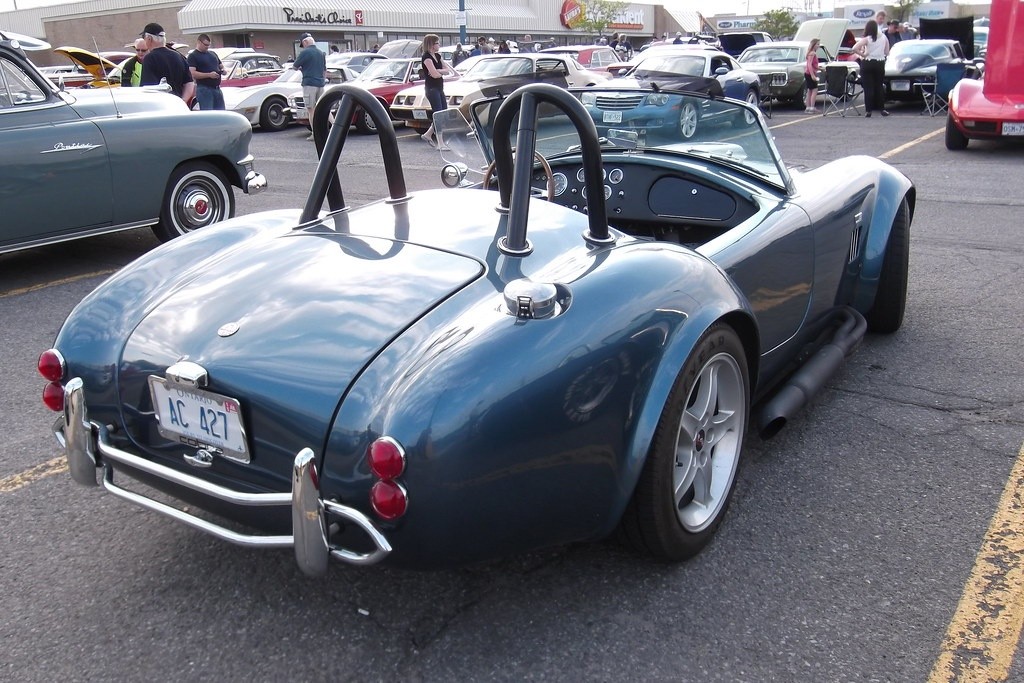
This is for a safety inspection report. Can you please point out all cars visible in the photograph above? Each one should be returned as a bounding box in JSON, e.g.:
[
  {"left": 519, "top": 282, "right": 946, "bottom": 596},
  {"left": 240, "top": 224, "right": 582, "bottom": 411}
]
[
  {"left": 0, "top": 35, "right": 270, "bottom": 264},
  {"left": 1, "top": 0, "right": 1024, "bottom": 155}
]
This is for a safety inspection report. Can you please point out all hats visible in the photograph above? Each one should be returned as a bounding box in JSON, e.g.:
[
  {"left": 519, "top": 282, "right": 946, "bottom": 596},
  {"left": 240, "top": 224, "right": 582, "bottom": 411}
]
[
  {"left": 675, "top": 32, "right": 682, "bottom": 35},
  {"left": 476, "top": 37, "right": 486, "bottom": 42},
  {"left": 498, "top": 39, "right": 506, "bottom": 45},
  {"left": 139, "top": 23, "right": 166, "bottom": 37},
  {"left": 887, "top": 19, "right": 899, "bottom": 25},
  {"left": 299, "top": 32, "right": 312, "bottom": 47}
]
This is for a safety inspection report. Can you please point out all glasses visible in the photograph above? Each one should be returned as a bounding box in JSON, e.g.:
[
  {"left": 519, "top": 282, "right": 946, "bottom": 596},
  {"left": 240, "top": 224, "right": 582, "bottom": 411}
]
[
  {"left": 433, "top": 42, "right": 440, "bottom": 45},
  {"left": 134, "top": 47, "right": 148, "bottom": 53},
  {"left": 199, "top": 41, "right": 211, "bottom": 47},
  {"left": 142, "top": 35, "right": 146, "bottom": 39}
]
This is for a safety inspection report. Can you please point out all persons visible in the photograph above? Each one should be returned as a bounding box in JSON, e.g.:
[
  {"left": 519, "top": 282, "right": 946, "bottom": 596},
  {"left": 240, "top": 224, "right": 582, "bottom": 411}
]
[
  {"left": 852, "top": 20, "right": 889, "bottom": 117},
  {"left": 187, "top": 34, "right": 227, "bottom": 110},
  {"left": 900, "top": 22, "right": 913, "bottom": 40},
  {"left": 498, "top": 40, "right": 511, "bottom": 54},
  {"left": 673, "top": 31, "right": 683, "bottom": 44},
  {"left": 292, "top": 33, "right": 335, "bottom": 141},
  {"left": 121, "top": 39, "right": 151, "bottom": 87},
  {"left": 139, "top": 23, "right": 194, "bottom": 102},
  {"left": 525, "top": 35, "right": 531, "bottom": 42},
  {"left": 287, "top": 55, "right": 295, "bottom": 63},
  {"left": 371, "top": 44, "right": 380, "bottom": 53},
  {"left": 470, "top": 44, "right": 481, "bottom": 57},
  {"left": 884, "top": 19, "right": 902, "bottom": 47},
  {"left": 876, "top": 10, "right": 886, "bottom": 32},
  {"left": 330, "top": 45, "right": 339, "bottom": 55},
  {"left": 452, "top": 42, "right": 468, "bottom": 70},
  {"left": 596, "top": 32, "right": 631, "bottom": 53},
  {"left": 804, "top": 38, "right": 823, "bottom": 114},
  {"left": 478, "top": 36, "right": 495, "bottom": 54},
  {"left": 421, "top": 34, "right": 451, "bottom": 151}
]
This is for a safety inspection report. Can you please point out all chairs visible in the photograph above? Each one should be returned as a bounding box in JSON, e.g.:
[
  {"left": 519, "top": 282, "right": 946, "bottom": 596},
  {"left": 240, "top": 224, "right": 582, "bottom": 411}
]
[
  {"left": 913, "top": 63, "right": 966, "bottom": 117},
  {"left": 758, "top": 74, "right": 773, "bottom": 119},
  {"left": 823, "top": 66, "right": 863, "bottom": 118}
]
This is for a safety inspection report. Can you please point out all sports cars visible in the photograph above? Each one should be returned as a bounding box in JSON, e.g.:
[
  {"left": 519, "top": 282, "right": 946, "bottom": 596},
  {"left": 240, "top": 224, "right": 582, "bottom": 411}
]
[{"left": 39, "top": 81, "right": 920, "bottom": 582}]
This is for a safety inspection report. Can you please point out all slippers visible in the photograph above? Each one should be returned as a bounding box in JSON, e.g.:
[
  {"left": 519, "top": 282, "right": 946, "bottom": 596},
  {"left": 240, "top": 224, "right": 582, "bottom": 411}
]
[
  {"left": 434, "top": 143, "right": 449, "bottom": 152},
  {"left": 421, "top": 133, "right": 436, "bottom": 147}
]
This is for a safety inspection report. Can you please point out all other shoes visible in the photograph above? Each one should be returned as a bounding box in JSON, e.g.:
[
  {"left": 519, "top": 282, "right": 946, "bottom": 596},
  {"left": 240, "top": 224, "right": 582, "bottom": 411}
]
[
  {"left": 881, "top": 111, "right": 891, "bottom": 116},
  {"left": 805, "top": 108, "right": 809, "bottom": 113},
  {"left": 306, "top": 133, "right": 315, "bottom": 141},
  {"left": 809, "top": 107, "right": 820, "bottom": 114},
  {"left": 865, "top": 112, "right": 871, "bottom": 118}
]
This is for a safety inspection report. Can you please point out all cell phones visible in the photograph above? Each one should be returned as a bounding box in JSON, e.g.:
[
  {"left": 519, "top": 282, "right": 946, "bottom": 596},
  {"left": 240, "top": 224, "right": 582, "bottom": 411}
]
[{"left": 217, "top": 71, "right": 223, "bottom": 75}]
